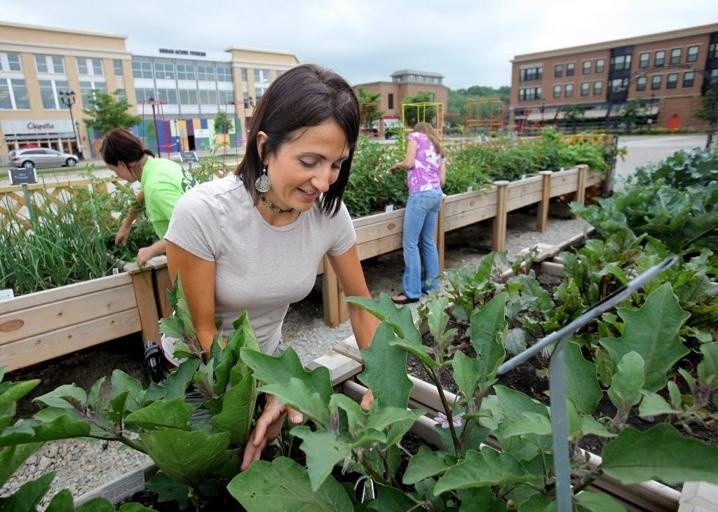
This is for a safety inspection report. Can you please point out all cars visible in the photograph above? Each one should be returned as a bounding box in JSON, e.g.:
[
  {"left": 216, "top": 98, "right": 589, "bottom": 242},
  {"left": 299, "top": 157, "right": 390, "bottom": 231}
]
[
  {"left": 372, "top": 118, "right": 402, "bottom": 138},
  {"left": 11, "top": 148, "right": 78, "bottom": 169}
]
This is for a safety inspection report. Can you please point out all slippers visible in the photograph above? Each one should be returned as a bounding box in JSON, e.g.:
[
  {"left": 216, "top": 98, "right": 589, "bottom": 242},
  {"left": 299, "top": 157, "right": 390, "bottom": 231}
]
[
  {"left": 391, "top": 293, "right": 419, "bottom": 304},
  {"left": 421, "top": 290, "right": 429, "bottom": 294}
]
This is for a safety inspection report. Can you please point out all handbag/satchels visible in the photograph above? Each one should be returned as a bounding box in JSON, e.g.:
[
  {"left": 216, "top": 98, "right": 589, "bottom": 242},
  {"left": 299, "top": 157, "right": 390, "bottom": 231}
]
[{"left": 144, "top": 343, "right": 160, "bottom": 376}]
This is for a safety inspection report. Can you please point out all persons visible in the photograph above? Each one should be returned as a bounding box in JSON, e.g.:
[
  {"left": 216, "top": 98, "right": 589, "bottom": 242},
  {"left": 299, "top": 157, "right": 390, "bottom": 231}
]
[
  {"left": 160, "top": 64, "right": 381, "bottom": 471},
  {"left": 390, "top": 122, "right": 446, "bottom": 304},
  {"left": 98, "top": 128, "right": 200, "bottom": 267}
]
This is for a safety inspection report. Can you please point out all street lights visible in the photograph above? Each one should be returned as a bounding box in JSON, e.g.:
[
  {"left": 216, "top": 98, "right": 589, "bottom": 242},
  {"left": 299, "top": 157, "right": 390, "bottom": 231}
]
[
  {"left": 59, "top": 90, "right": 82, "bottom": 159},
  {"left": 223, "top": 101, "right": 235, "bottom": 163},
  {"left": 149, "top": 98, "right": 160, "bottom": 159}
]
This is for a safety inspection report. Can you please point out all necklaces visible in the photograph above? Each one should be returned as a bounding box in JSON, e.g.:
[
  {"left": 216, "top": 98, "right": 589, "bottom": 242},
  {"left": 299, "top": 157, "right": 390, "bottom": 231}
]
[{"left": 259, "top": 193, "right": 294, "bottom": 214}]
